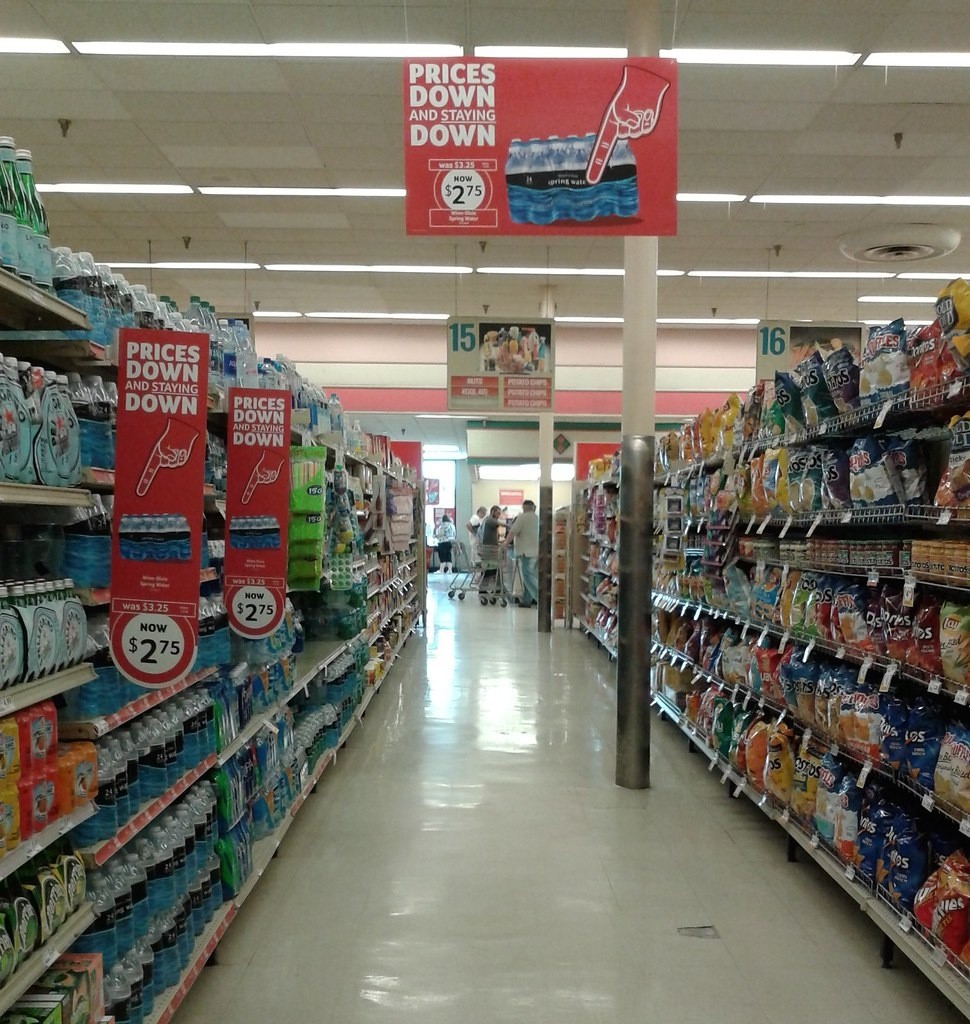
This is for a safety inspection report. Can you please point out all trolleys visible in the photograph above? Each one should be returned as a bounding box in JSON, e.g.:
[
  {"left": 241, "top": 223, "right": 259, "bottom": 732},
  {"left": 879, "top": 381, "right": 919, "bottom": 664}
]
[
  {"left": 478, "top": 542, "right": 516, "bottom": 606},
  {"left": 446, "top": 539, "right": 484, "bottom": 601}
]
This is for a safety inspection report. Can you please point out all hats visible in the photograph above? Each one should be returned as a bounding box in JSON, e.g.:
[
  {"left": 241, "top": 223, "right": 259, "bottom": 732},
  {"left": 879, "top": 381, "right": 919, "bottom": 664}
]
[{"left": 524, "top": 501, "right": 534, "bottom": 505}]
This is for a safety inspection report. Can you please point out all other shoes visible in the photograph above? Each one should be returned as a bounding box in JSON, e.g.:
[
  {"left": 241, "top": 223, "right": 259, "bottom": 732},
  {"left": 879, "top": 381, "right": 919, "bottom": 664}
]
[
  {"left": 491, "top": 590, "right": 505, "bottom": 594},
  {"left": 434, "top": 570, "right": 444, "bottom": 574},
  {"left": 518, "top": 604, "right": 530, "bottom": 608},
  {"left": 447, "top": 570, "right": 452, "bottom": 573},
  {"left": 478, "top": 591, "right": 487, "bottom": 594}
]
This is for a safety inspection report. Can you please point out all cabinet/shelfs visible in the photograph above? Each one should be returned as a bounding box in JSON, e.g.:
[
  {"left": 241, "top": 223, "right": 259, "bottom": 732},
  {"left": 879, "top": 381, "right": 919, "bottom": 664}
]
[
  {"left": 553, "top": 379, "right": 970, "bottom": 1024},
  {"left": 0, "top": 266, "right": 423, "bottom": 1024}
]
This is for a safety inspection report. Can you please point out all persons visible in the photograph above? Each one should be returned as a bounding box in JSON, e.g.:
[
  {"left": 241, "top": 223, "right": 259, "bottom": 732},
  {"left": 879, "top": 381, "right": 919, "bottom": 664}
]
[
  {"left": 434, "top": 514, "right": 456, "bottom": 575},
  {"left": 466, "top": 500, "right": 538, "bottom": 608}
]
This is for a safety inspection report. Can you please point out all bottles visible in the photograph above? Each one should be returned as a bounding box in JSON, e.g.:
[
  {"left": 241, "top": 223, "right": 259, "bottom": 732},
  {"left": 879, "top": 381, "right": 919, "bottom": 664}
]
[
  {"left": 79, "top": 779, "right": 223, "bottom": 1024},
  {"left": 320, "top": 638, "right": 366, "bottom": 746},
  {"left": 53, "top": 246, "right": 257, "bottom": 396},
  {"left": 0, "top": 134, "right": 52, "bottom": 291},
  {"left": 259, "top": 353, "right": 366, "bottom": 456},
  {"left": 70, "top": 591, "right": 233, "bottom": 847},
  {"left": 0, "top": 350, "right": 225, "bottom": 586},
  {"left": 504, "top": 130, "right": 639, "bottom": 225},
  {"left": 233, "top": 596, "right": 305, "bottom": 734},
  {"left": 291, "top": 697, "right": 338, "bottom": 788},
  {"left": 0, "top": 577, "right": 88, "bottom": 691},
  {"left": 492, "top": 327, "right": 546, "bottom": 371}
]
